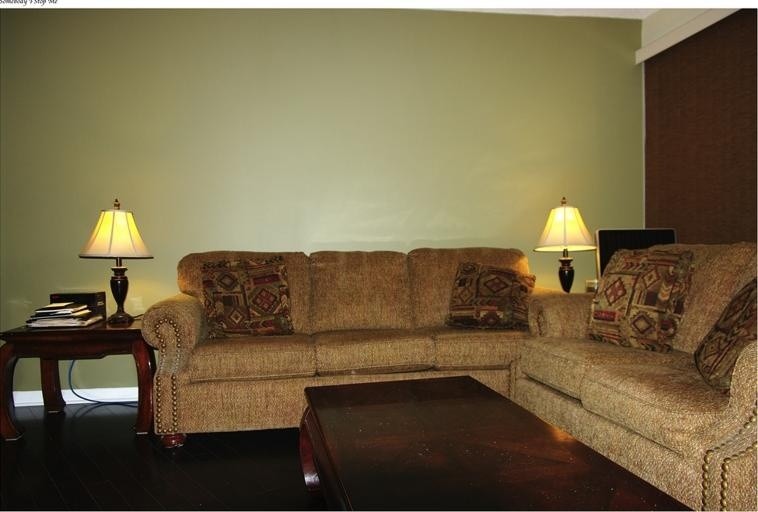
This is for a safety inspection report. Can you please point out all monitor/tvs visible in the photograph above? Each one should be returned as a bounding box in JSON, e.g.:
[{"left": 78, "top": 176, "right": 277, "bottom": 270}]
[{"left": 594, "top": 226, "right": 678, "bottom": 280}]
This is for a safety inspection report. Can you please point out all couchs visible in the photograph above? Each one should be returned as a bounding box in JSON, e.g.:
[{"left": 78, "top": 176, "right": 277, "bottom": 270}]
[
  {"left": 505, "top": 241, "right": 757, "bottom": 511},
  {"left": 140, "top": 245, "right": 565, "bottom": 476}
]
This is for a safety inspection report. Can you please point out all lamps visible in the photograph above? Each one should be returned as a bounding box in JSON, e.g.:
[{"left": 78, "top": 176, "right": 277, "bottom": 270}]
[
  {"left": 78, "top": 198, "right": 154, "bottom": 328},
  {"left": 531, "top": 197, "right": 596, "bottom": 293}
]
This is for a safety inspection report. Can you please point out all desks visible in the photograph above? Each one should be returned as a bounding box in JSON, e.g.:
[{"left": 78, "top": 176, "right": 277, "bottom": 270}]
[{"left": 0, "top": 320, "right": 158, "bottom": 443}]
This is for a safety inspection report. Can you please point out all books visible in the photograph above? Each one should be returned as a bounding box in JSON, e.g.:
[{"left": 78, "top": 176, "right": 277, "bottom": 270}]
[{"left": 24, "top": 301, "right": 103, "bottom": 329}]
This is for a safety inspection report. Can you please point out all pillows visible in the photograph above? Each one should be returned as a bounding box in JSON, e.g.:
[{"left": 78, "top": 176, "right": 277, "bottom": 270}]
[
  {"left": 587, "top": 248, "right": 693, "bottom": 355},
  {"left": 693, "top": 274, "right": 757, "bottom": 393},
  {"left": 446, "top": 256, "right": 538, "bottom": 335},
  {"left": 195, "top": 254, "right": 296, "bottom": 338}
]
